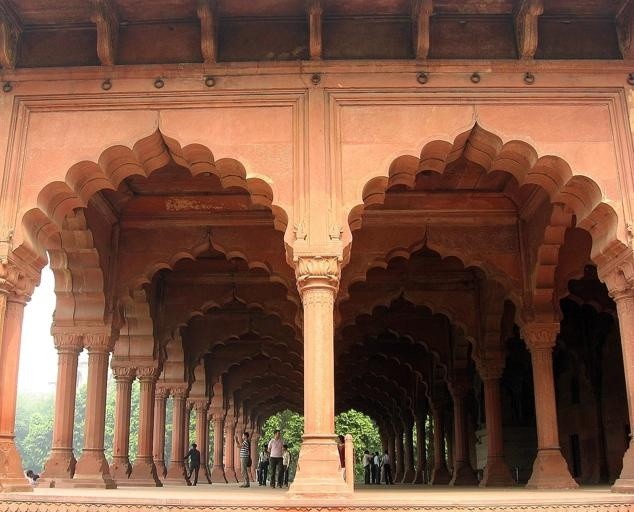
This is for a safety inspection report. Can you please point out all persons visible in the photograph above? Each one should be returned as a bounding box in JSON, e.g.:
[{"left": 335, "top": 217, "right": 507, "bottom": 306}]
[
  {"left": 258, "top": 444, "right": 270, "bottom": 486},
  {"left": 27, "top": 471, "right": 40, "bottom": 481},
  {"left": 268, "top": 430, "right": 285, "bottom": 489},
  {"left": 184, "top": 444, "right": 200, "bottom": 486},
  {"left": 336, "top": 435, "right": 345, "bottom": 481},
  {"left": 282, "top": 446, "right": 290, "bottom": 486},
  {"left": 235, "top": 432, "right": 251, "bottom": 488},
  {"left": 363, "top": 451, "right": 395, "bottom": 485}
]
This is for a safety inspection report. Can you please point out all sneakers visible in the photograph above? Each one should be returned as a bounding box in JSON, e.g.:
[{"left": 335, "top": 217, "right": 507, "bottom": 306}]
[
  {"left": 259, "top": 483, "right": 266, "bottom": 486},
  {"left": 239, "top": 485, "right": 250, "bottom": 487},
  {"left": 272, "top": 484, "right": 288, "bottom": 488}
]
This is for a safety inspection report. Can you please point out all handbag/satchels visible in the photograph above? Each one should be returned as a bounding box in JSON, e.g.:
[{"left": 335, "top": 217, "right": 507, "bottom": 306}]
[{"left": 247, "top": 457, "right": 252, "bottom": 467}]
